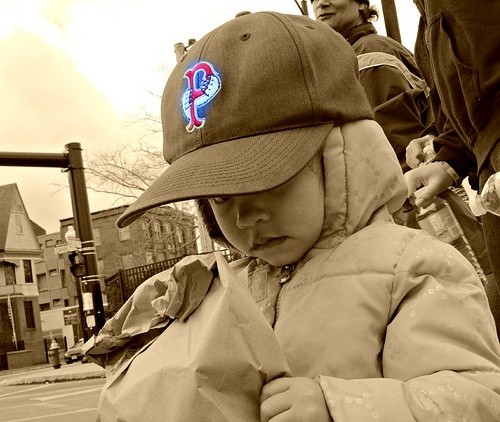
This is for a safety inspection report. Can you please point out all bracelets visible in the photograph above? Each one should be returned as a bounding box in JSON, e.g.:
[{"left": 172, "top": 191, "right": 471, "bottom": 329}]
[{"left": 436, "top": 161, "right": 461, "bottom": 189}]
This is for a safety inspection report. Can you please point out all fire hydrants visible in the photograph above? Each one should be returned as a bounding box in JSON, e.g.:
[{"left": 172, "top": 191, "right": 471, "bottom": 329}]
[{"left": 48, "top": 339, "right": 62, "bottom": 369}]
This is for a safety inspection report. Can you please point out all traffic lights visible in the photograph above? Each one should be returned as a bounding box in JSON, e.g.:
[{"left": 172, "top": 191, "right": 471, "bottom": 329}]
[{"left": 69, "top": 252, "right": 86, "bottom": 278}]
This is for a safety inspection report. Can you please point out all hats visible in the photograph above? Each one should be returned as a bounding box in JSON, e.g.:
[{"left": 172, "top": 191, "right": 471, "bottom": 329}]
[{"left": 114, "top": 11, "right": 375, "bottom": 229}]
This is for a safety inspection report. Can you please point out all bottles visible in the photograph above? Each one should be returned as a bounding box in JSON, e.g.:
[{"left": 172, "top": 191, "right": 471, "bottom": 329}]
[{"left": 413, "top": 186, "right": 489, "bottom": 290}]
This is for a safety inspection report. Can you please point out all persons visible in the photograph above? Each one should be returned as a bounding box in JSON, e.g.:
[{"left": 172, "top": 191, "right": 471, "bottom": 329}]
[
  {"left": 310, "top": 0, "right": 500, "bottom": 296},
  {"left": 114, "top": 12, "right": 500, "bottom": 422}
]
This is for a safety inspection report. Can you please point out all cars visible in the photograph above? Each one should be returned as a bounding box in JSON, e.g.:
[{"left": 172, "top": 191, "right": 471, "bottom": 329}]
[{"left": 64, "top": 338, "right": 84, "bottom": 365}]
